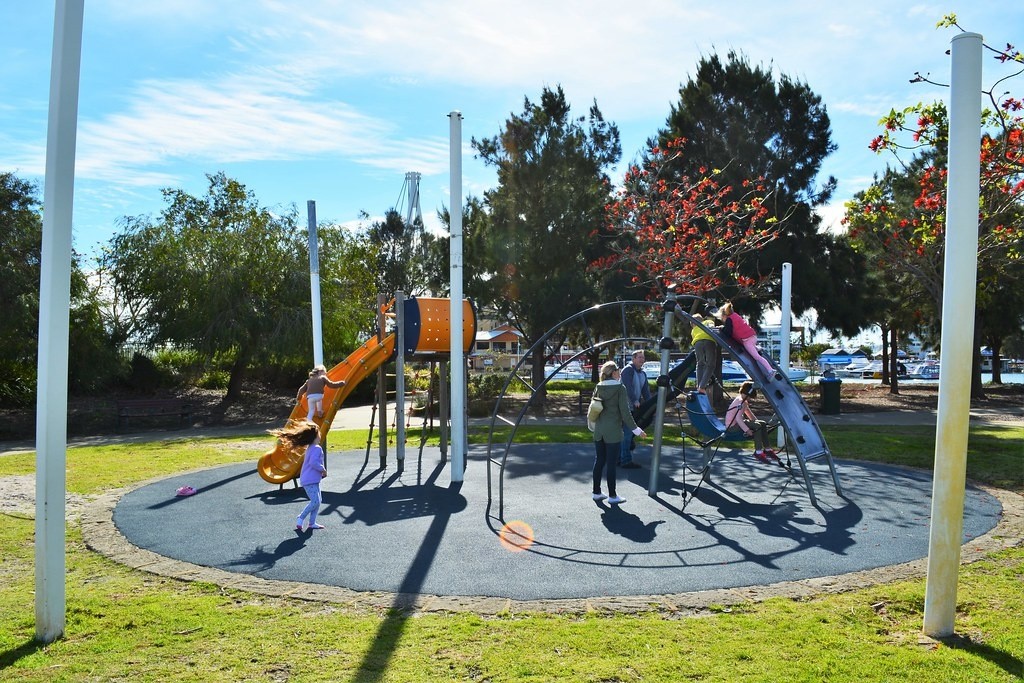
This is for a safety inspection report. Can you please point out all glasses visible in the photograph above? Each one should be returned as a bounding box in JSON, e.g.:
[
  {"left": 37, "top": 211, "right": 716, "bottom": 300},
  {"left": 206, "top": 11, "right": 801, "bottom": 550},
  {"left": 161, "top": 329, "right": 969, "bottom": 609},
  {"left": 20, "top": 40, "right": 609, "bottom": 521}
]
[{"left": 615, "top": 368, "right": 619, "bottom": 371}]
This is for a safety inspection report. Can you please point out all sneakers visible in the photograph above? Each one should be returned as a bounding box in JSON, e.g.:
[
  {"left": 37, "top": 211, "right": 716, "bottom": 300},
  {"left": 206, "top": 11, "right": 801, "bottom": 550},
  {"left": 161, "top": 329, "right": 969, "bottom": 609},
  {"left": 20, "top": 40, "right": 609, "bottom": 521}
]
[
  {"left": 754, "top": 450, "right": 768, "bottom": 463},
  {"left": 763, "top": 449, "right": 778, "bottom": 459}
]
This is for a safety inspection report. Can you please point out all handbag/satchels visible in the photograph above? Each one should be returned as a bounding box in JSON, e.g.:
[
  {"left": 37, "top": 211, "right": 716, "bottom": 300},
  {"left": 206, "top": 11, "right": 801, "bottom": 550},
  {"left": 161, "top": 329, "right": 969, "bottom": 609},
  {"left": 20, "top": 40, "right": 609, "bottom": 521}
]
[{"left": 587, "top": 393, "right": 604, "bottom": 421}]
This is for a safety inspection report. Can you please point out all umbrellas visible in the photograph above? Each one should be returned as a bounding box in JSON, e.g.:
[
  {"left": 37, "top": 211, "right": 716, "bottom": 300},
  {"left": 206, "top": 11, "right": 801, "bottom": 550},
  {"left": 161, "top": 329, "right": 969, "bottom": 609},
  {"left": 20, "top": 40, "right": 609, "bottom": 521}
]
[{"left": 872, "top": 347, "right": 906, "bottom": 356}]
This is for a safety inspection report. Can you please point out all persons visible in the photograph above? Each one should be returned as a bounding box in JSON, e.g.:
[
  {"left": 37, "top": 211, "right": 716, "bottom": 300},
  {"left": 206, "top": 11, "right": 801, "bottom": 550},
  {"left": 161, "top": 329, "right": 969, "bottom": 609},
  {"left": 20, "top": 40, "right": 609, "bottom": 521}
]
[
  {"left": 716, "top": 303, "right": 776, "bottom": 383},
  {"left": 619, "top": 349, "right": 652, "bottom": 468},
  {"left": 265, "top": 417, "right": 327, "bottom": 530},
  {"left": 296, "top": 364, "right": 345, "bottom": 422},
  {"left": 725, "top": 381, "right": 781, "bottom": 466},
  {"left": 689, "top": 313, "right": 717, "bottom": 394},
  {"left": 587, "top": 360, "right": 646, "bottom": 504}
]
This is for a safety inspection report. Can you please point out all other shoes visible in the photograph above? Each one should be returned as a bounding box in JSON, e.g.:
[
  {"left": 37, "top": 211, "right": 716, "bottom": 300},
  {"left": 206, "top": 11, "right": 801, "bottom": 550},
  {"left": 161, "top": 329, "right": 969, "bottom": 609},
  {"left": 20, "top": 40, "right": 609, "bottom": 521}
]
[
  {"left": 767, "top": 370, "right": 777, "bottom": 382},
  {"left": 622, "top": 462, "right": 640, "bottom": 468},
  {"left": 177, "top": 486, "right": 196, "bottom": 495},
  {"left": 593, "top": 492, "right": 607, "bottom": 500},
  {"left": 608, "top": 496, "right": 626, "bottom": 504},
  {"left": 698, "top": 388, "right": 706, "bottom": 394}
]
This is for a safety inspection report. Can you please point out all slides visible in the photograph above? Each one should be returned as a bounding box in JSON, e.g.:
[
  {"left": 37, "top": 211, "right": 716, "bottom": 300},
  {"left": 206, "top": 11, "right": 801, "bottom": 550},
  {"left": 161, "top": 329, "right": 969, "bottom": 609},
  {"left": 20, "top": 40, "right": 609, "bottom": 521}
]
[{"left": 257, "top": 330, "right": 401, "bottom": 483}]
